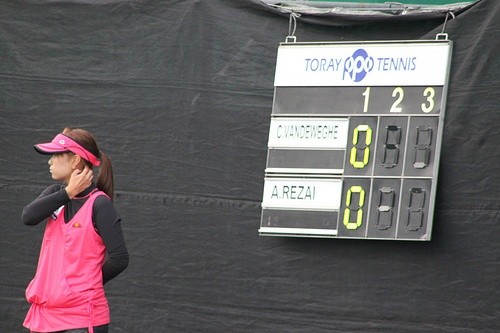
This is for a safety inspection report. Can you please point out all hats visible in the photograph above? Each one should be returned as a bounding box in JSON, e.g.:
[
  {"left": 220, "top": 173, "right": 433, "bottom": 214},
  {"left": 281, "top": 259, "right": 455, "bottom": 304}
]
[{"left": 32, "top": 133, "right": 101, "bottom": 167}]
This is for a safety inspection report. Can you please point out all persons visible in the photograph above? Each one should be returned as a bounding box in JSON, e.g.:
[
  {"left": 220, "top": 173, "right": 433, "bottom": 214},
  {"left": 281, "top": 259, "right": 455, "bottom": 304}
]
[{"left": 19, "top": 126, "right": 131, "bottom": 333}]
